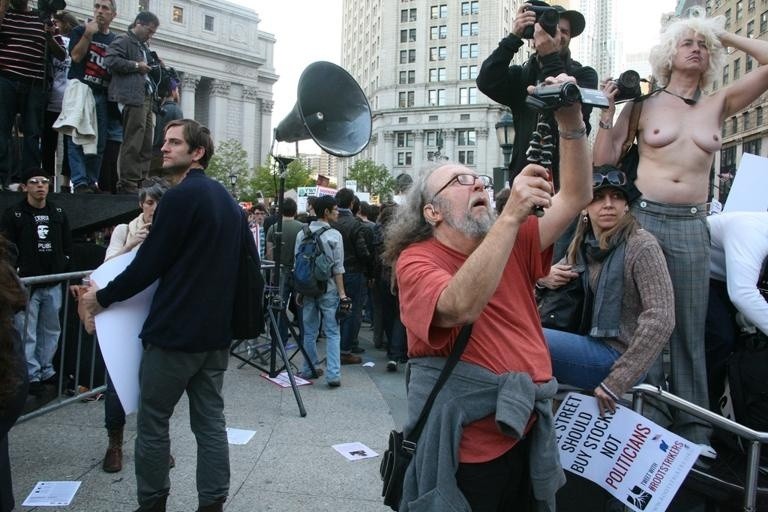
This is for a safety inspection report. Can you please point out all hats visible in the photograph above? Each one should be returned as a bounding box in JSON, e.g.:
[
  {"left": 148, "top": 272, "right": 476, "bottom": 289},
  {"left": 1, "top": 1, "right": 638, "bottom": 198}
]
[
  {"left": 23, "top": 167, "right": 52, "bottom": 183},
  {"left": 591, "top": 164, "right": 643, "bottom": 203},
  {"left": 549, "top": 4, "right": 586, "bottom": 38}
]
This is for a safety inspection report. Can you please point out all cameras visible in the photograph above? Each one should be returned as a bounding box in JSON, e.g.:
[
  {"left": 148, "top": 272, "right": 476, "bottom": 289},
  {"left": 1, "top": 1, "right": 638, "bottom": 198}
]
[
  {"left": 521, "top": 6, "right": 559, "bottom": 38},
  {"left": 157, "top": 105, "right": 167, "bottom": 116},
  {"left": 527, "top": 83, "right": 610, "bottom": 113},
  {"left": 611, "top": 70, "right": 642, "bottom": 102}
]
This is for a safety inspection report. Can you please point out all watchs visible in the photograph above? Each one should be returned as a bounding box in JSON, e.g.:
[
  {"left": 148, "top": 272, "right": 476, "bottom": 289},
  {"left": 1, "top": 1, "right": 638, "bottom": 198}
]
[{"left": 598, "top": 120, "right": 612, "bottom": 129}]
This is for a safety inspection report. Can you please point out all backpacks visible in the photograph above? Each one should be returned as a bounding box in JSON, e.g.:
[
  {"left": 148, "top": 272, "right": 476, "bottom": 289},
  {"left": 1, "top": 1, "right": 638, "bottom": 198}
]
[{"left": 290, "top": 223, "right": 335, "bottom": 297}]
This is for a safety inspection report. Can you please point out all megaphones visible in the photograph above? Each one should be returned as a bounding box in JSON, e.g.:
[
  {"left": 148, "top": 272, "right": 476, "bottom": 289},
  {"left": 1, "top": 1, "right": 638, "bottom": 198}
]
[{"left": 272, "top": 60, "right": 372, "bottom": 159}]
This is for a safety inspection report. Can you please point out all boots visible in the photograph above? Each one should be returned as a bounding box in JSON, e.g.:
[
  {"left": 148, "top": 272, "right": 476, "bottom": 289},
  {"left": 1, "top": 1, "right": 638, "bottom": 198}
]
[
  {"left": 191, "top": 496, "right": 226, "bottom": 512},
  {"left": 102, "top": 421, "right": 124, "bottom": 472},
  {"left": 131, "top": 494, "right": 170, "bottom": 512}
]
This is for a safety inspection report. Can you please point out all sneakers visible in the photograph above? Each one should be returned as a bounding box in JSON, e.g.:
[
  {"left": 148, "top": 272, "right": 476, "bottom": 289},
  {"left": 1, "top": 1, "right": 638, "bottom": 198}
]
[
  {"left": 45, "top": 371, "right": 70, "bottom": 387},
  {"left": 374, "top": 341, "right": 384, "bottom": 348},
  {"left": 328, "top": 379, "right": 341, "bottom": 387},
  {"left": 302, "top": 368, "right": 323, "bottom": 379},
  {"left": 688, "top": 439, "right": 718, "bottom": 461},
  {"left": 384, "top": 360, "right": 397, "bottom": 370},
  {"left": 351, "top": 345, "right": 364, "bottom": 353},
  {"left": 28, "top": 380, "right": 49, "bottom": 396},
  {"left": 58, "top": 183, "right": 163, "bottom": 195},
  {"left": 338, "top": 353, "right": 362, "bottom": 365}
]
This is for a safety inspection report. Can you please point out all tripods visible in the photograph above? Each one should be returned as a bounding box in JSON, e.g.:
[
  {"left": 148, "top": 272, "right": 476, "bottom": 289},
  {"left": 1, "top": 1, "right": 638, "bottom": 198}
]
[{"left": 228, "top": 169, "right": 318, "bottom": 417}]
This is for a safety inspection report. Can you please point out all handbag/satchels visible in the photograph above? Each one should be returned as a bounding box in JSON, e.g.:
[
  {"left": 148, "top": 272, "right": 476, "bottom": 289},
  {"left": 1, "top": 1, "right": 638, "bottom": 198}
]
[
  {"left": 615, "top": 142, "right": 641, "bottom": 201},
  {"left": 378, "top": 429, "right": 412, "bottom": 512},
  {"left": 232, "top": 201, "right": 266, "bottom": 341},
  {"left": 532, "top": 264, "right": 594, "bottom": 335}
]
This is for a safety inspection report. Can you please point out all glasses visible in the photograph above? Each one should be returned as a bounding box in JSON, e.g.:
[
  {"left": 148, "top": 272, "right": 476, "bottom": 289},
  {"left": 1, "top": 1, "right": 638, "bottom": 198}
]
[
  {"left": 28, "top": 178, "right": 50, "bottom": 184},
  {"left": 430, "top": 174, "right": 492, "bottom": 207},
  {"left": 590, "top": 170, "right": 629, "bottom": 190}
]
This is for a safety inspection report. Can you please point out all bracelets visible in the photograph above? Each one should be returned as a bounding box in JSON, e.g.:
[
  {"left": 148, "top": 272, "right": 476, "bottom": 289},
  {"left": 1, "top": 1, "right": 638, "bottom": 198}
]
[{"left": 559, "top": 123, "right": 588, "bottom": 140}]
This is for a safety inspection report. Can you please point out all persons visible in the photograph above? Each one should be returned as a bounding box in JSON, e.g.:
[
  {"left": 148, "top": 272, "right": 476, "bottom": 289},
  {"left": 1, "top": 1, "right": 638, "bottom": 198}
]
[
  {"left": 591, "top": 4, "right": 768, "bottom": 473},
  {"left": 375, "top": 70, "right": 597, "bottom": 510},
  {"left": 0, "top": 1, "right": 419, "bottom": 511},
  {"left": 704, "top": 211, "right": 768, "bottom": 473},
  {"left": 532, "top": 163, "right": 678, "bottom": 414},
  {"left": 476, "top": 1, "right": 596, "bottom": 268}
]
